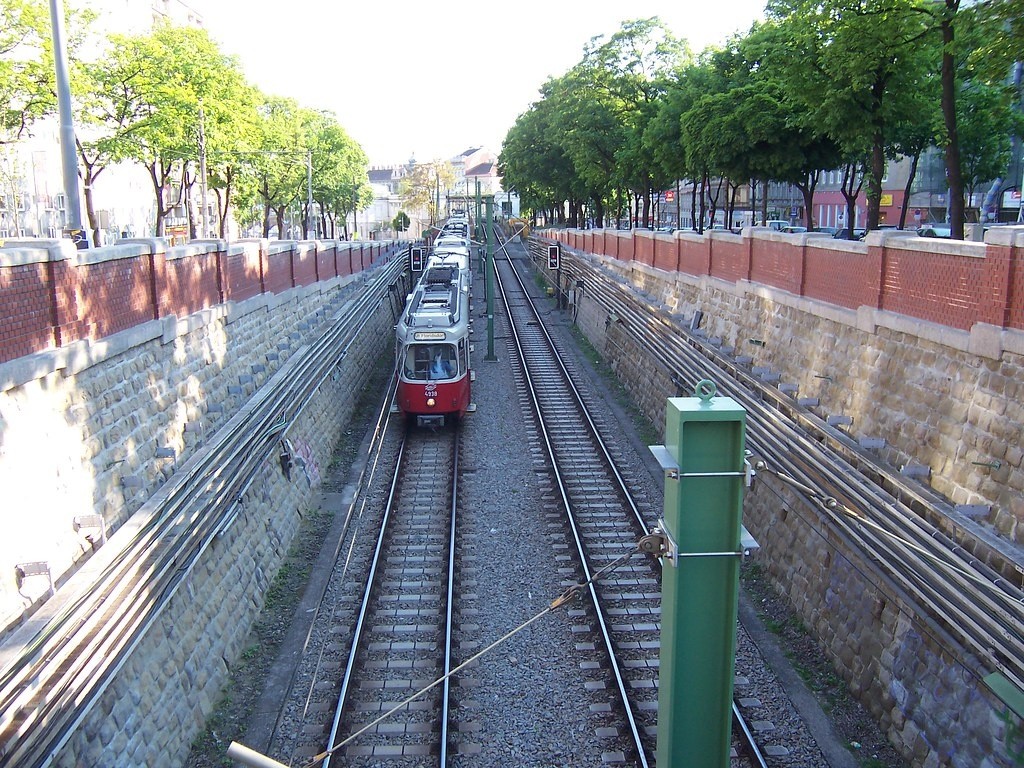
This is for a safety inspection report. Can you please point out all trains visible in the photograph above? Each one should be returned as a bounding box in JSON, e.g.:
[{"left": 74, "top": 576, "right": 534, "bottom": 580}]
[{"left": 394, "top": 214, "right": 476, "bottom": 428}]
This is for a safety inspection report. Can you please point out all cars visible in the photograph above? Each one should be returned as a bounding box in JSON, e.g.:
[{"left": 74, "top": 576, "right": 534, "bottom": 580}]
[{"left": 584, "top": 218, "right": 1024, "bottom": 241}]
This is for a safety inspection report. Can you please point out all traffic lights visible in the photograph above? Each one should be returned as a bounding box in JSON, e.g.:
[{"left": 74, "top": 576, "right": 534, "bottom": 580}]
[
  {"left": 547, "top": 246, "right": 560, "bottom": 270},
  {"left": 411, "top": 248, "right": 423, "bottom": 272}
]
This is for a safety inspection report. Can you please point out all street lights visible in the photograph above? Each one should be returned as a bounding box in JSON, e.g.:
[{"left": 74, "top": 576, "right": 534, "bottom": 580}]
[{"left": 31, "top": 151, "right": 45, "bottom": 223}]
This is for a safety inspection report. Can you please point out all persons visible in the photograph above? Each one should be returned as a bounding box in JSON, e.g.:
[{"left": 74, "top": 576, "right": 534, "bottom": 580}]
[{"left": 429, "top": 350, "right": 455, "bottom": 379}]
